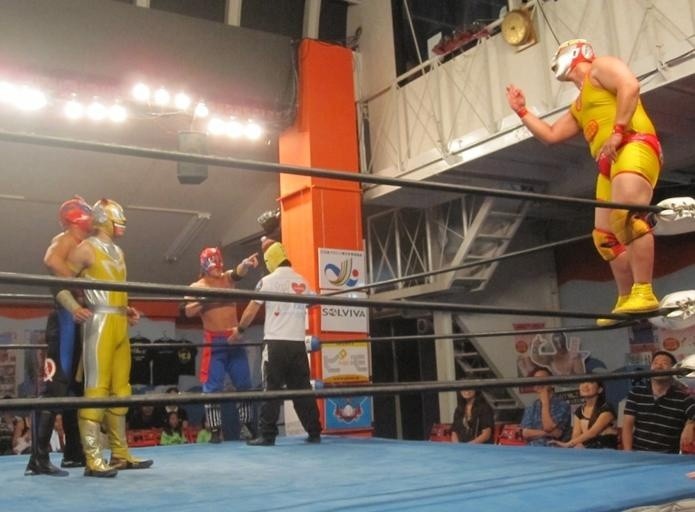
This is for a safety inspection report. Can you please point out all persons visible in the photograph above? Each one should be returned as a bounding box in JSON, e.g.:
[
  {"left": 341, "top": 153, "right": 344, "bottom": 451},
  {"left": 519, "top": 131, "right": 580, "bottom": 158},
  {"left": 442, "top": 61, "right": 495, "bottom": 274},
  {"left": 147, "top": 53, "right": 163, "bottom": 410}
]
[
  {"left": 546, "top": 381, "right": 618, "bottom": 450},
  {"left": 621, "top": 351, "right": 695, "bottom": 454},
  {"left": 23, "top": 194, "right": 96, "bottom": 476},
  {"left": 505, "top": 38, "right": 664, "bottom": 328},
  {"left": 513, "top": 366, "right": 571, "bottom": 447},
  {"left": 531, "top": 332, "right": 589, "bottom": 376},
  {"left": 450, "top": 376, "right": 494, "bottom": 443},
  {"left": 52, "top": 198, "right": 154, "bottom": 477},
  {"left": 226, "top": 236, "right": 322, "bottom": 445},
  {"left": 185, "top": 247, "right": 259, "bottom": 444},
  {"left": 0, "top": 387, "right": 189, "bottom": 455}
]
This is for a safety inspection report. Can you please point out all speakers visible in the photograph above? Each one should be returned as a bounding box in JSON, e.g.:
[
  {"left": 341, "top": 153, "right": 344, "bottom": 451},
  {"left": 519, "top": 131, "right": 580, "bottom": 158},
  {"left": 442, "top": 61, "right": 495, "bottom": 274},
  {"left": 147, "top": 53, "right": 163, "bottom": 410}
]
[{"left": 177, "top": 132, "right": 208, "bottom": 185}]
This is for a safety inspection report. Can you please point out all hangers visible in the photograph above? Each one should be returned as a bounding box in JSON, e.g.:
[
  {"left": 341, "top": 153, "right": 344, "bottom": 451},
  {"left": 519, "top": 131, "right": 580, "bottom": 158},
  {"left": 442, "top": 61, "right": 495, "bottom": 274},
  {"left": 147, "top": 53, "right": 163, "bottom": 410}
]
[
  {"left": 135, "top": 331, "right": 141, "bottom": 338},
  {"left": 160, "top": 330, "right": 171, "bottom": 341}
]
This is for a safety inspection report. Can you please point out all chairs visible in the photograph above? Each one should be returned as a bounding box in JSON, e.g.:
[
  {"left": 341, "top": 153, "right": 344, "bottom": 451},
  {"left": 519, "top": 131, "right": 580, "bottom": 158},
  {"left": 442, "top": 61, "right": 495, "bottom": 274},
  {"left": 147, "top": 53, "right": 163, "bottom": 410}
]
[
  {"left": 127, "top": 427, "right": 199, "bottom": 448},
  {"left": 427, "top": 422, "right": 452, "bottom": 441},
  {"left": 498, "top": 424, "right": 527, "bottom": 445},
  {"left": 494, "top": 424, "right": 503, "bottom": 445}
]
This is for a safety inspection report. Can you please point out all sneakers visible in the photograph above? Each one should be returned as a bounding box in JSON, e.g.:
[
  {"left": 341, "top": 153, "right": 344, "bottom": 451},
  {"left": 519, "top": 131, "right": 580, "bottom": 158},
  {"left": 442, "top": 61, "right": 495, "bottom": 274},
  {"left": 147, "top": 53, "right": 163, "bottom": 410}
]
[
  {"left": 596, "top": 285, "right": 660, "bottom": 326},
  {"left": 305, "top": 429, "right": 322, "bottom": 445},
  {"left": 209, "top": 425, "right": 221, "bottom": 445},
  {"left": 240, "top": 426, "right": 276, "bottom": 447}
]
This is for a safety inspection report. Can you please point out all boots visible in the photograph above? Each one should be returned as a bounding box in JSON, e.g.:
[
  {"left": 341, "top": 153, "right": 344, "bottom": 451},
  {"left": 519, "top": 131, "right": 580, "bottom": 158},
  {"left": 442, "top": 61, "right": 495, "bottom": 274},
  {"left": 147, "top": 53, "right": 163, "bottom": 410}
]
[{"left": 25, "top": 407, "right": 154, "bottom": 477}]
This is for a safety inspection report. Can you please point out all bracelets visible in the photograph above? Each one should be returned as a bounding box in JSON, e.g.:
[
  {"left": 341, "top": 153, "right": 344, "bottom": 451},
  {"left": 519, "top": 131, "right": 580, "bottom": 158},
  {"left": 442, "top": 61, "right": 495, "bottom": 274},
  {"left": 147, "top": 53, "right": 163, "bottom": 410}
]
[
  {"left": 237, "top": 326, "right": 245, "bottom": 334},
  {"left": 615, "top": 125, "right": 625, "bottom": 132},
  {"left": 518, "top": 108, "right": 528, "bottom": 117}
]
